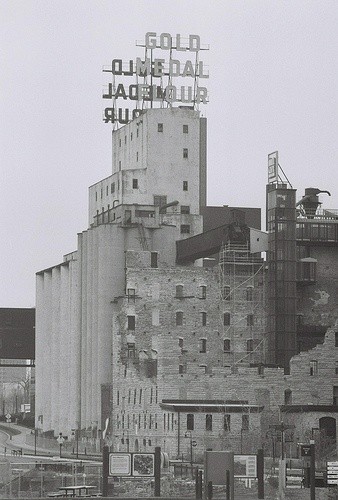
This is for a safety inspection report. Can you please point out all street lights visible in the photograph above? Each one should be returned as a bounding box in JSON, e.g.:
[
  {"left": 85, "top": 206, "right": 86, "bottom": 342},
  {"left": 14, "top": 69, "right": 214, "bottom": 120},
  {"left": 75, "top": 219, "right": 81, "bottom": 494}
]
[
  {"left": 312, "top": 427, "right": 319, "bottom": 439},
  {"left": 185, "top": 430, "right": 193, "bottom": 463}
]
